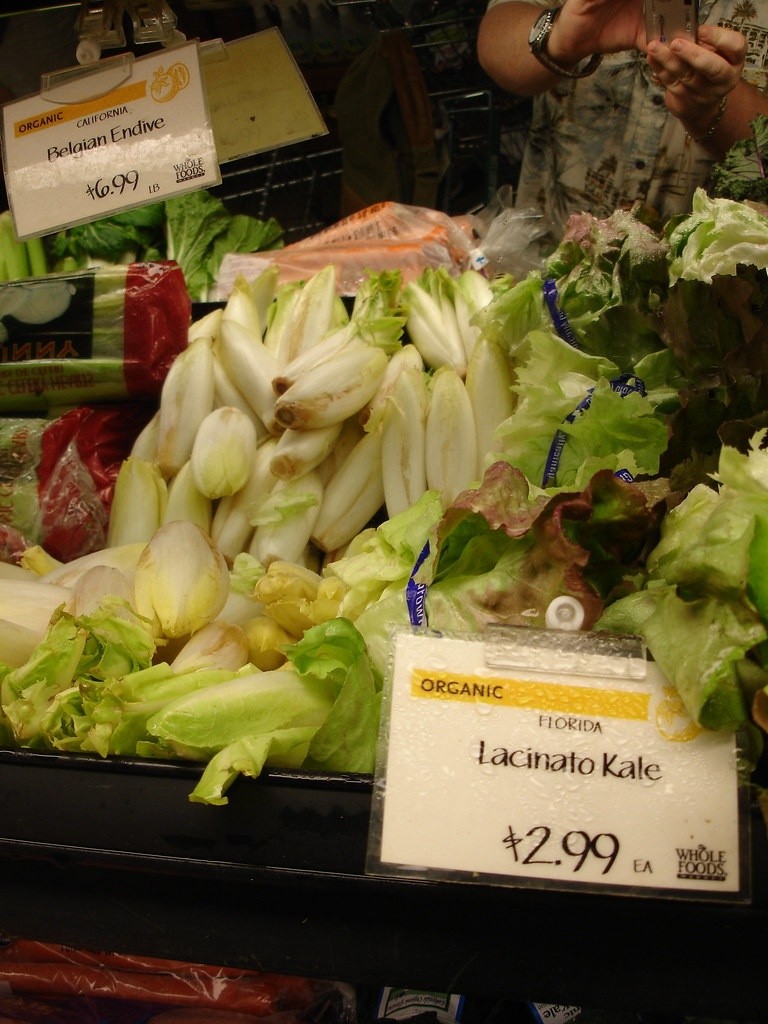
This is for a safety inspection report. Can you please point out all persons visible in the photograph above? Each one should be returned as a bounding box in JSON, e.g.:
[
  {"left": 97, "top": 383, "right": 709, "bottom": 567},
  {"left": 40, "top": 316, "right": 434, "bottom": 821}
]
[{"left": 477, "top": 0, "right": 768, "bottom": 254}]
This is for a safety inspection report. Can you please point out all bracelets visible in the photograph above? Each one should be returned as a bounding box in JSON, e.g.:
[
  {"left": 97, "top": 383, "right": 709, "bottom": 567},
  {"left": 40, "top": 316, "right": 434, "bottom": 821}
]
[{"left": 683, "top": 94, "right": 728, "bottom": 145}]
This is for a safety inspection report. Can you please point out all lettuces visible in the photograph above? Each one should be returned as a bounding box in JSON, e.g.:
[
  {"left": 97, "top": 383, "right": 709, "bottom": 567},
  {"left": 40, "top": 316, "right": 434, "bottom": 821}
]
[{"left": 283, "top": 184, "right": 768, "bottom": 833}]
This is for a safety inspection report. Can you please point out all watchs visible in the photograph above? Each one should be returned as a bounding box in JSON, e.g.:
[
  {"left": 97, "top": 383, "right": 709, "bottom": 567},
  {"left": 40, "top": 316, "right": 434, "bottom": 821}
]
[{"left": 527, "top": 3, "right": 605, "bottom": 83}]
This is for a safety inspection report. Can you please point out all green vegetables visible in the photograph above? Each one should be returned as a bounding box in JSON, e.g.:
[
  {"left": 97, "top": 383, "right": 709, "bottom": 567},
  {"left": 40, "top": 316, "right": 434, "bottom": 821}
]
[{"left": 0, "top": 194, "right": 507, "bottom": 806}]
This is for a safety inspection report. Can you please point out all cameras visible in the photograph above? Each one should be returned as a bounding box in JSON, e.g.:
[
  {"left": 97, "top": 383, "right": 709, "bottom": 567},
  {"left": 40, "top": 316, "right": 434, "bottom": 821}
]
[{"left": 641, "top": 0, "right": 699, "bottom": 46}]
